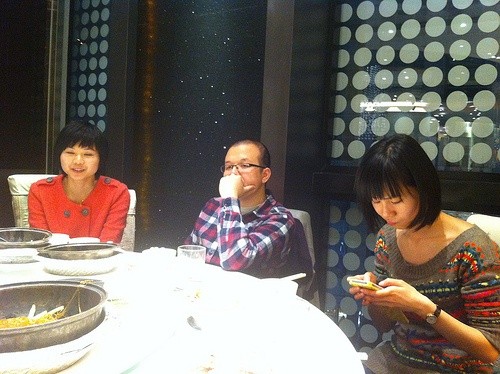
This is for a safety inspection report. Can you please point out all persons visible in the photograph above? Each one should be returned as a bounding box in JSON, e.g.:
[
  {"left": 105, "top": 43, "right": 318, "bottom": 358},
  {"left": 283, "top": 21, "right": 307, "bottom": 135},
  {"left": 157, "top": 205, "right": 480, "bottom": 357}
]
[
  {"left": 347, "top": 133, "right": 500, "bottom": 374},
  {"left": 27, "top": 120, "right": 131, "bottom": 247},
  {"left": 184, "top": 140, "right": 312, "bottom": 288}
]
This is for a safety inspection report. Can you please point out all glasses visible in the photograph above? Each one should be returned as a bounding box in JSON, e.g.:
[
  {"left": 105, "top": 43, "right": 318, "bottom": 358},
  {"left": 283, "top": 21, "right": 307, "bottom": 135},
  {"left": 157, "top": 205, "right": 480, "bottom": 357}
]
[{"left": 220, "top": 163, "right": 265, "bottom": 172}]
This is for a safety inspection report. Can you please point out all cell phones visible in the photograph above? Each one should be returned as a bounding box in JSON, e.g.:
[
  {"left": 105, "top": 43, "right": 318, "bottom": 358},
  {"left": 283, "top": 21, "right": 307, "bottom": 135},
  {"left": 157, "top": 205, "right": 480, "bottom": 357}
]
[{"left": 348, "top": 279, "right": 383, "bottom": 291}]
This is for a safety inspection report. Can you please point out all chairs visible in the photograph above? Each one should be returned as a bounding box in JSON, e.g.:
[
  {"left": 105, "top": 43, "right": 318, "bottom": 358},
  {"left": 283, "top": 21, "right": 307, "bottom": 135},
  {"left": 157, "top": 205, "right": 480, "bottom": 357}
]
[
  {"left": 466, "top": 213, "right": 500, "bottom": 247},
  {"left": 286, "top": 208, "right": 320, "bottom": 312},
  {"left": 7, "top": 173, "right": 137, "bottom": 253}
]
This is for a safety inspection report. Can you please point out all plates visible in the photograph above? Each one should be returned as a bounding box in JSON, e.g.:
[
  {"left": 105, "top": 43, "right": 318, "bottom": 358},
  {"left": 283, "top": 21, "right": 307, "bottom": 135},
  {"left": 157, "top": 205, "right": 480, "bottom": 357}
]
[{"left": 69, "top": 237, "right": 100, "bottom": 244}]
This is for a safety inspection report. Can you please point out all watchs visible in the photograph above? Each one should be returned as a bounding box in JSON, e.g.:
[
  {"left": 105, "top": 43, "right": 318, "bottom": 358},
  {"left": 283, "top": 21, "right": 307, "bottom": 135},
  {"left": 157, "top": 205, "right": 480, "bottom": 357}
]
[{"left": 425, "top": 304, "right": 443, "bottom": 324}]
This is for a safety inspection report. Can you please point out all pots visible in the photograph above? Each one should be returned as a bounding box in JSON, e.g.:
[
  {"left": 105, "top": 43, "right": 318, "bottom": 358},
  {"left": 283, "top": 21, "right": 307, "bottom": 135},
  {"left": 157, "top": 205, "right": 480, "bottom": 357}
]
[
  {"left": 38, "top": 243, "right": 120, "bottom": 259},
  {"left": 0, "top": 278, "right": 108, "bottom": 353},
  {"left": 0, "top": 227, "right": 53, "bottom": 249}
]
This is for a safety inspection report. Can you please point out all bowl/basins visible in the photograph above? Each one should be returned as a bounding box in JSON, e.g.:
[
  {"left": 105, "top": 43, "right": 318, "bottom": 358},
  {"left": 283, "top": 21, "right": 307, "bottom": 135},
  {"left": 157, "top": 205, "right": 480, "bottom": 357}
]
[
  {"left": 0, "top": 307, "right": 107, "bottom": 374},
  {"left": 37, "top": 250, "right": 123, "bottom": 276},
  {"left": 0, "top": 249, "right": 37, "bottom": 263}
]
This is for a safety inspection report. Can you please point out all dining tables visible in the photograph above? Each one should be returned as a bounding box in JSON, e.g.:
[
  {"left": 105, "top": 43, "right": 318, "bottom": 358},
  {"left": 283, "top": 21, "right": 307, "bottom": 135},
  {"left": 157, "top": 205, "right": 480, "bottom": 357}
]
[{"left": 0, "top": 229, "right": 366, "bottom": 374}]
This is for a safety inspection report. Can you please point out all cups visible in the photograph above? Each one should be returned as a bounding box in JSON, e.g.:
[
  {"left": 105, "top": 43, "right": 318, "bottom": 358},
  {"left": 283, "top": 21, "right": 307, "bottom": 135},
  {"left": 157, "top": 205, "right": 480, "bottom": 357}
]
[
  {"left": 178, "top": 245, "right": 206, "bottom": 282},
  {"left": 49, "top": 233, "right": 69, "bottom": 245}
]
